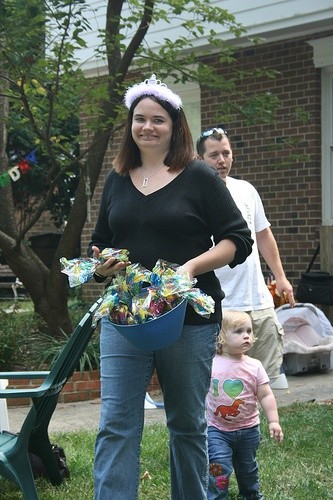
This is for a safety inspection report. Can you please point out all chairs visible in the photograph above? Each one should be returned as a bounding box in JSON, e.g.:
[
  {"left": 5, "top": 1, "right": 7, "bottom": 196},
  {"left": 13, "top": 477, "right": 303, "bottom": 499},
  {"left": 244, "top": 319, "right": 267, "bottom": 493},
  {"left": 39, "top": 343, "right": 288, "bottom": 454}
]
[{"left": 0, "top": 297, "right": 103, "bottom": 500}]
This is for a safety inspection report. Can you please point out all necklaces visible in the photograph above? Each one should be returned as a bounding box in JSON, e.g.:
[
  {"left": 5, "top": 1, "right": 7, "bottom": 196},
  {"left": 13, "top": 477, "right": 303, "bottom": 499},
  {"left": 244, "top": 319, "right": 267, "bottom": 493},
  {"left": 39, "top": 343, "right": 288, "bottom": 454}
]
[{"left": 138, "top": 163, "right": 164, "bottom": 188}]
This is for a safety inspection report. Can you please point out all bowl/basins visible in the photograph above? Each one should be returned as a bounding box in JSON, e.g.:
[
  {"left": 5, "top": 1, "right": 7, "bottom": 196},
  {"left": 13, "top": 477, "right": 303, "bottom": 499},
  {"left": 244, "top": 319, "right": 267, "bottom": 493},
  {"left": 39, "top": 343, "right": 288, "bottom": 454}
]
[{"left": 106, "top": 285, "right": 189, "bottom": 352}]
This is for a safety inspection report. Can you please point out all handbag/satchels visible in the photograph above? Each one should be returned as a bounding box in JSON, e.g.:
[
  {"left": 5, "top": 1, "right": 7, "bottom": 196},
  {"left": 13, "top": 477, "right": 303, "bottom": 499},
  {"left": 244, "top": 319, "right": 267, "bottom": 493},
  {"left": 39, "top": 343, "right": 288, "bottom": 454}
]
[{"left": 29, "top": 444, "right": 71, "bottom": 481}]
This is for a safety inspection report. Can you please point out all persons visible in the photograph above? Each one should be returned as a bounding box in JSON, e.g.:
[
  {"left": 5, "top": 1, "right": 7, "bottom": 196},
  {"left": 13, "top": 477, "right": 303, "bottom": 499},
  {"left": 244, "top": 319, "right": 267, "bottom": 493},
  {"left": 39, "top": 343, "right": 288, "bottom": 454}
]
[
  {"left": 201, "top": 309, "right": 284, "bottom": 500},
  {"left": 187, "top": 128, "right": 295, "bottom": 391},
  {"left": 88, "top": 74, "right": 255, "bottom": 500}
]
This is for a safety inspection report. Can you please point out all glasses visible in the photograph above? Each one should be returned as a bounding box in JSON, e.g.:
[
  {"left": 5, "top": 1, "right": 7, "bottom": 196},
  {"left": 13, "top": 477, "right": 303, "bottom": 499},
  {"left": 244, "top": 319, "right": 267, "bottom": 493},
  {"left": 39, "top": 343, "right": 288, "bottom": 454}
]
[{"left": 198, "top": 128, "right": 229, "bottom": 150}]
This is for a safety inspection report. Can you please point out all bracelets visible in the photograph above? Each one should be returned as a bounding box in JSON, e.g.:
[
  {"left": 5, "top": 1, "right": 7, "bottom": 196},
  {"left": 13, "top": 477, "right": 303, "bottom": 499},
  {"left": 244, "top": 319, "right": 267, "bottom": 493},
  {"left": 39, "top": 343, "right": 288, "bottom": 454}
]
[{"left": 94, "top": 272, "right": 103, "bottom": 277}]
